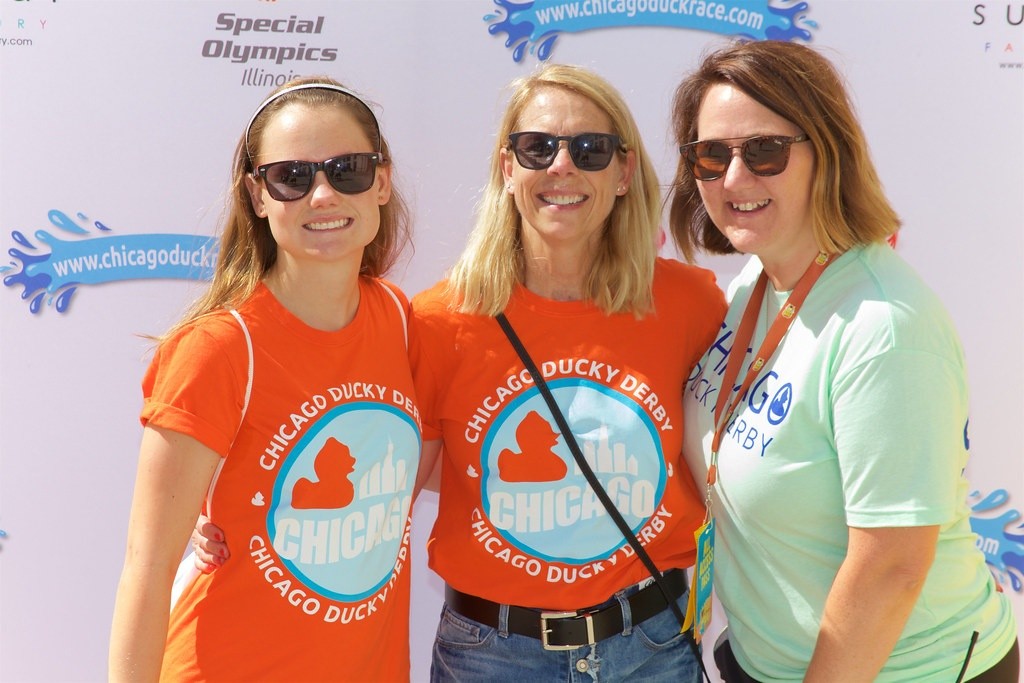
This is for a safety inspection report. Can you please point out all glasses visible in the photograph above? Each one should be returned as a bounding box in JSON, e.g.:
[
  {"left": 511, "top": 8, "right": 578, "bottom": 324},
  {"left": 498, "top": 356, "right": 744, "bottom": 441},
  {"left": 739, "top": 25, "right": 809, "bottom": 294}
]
[
  {"left": 508, "top": 130, "right": 626, "bottom": 172},
  {"left": 252, "top": 153, "right": 383, "bottom": 201},
  {"left": 679, "top": 134, "right": 811, "bottom": 182}
]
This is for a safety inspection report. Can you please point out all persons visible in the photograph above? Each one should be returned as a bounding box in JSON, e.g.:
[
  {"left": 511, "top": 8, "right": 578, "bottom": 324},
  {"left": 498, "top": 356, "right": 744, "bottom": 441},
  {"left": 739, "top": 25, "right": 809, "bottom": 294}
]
[
  {"left": 191, "top": 64, "right": 729, "bottom": 683},
  {"left": 108, "top": 76, "right": 444, "bottom": 683},
  {"left": 669, "top": 38, "right": 1020, "bottom": 683}
]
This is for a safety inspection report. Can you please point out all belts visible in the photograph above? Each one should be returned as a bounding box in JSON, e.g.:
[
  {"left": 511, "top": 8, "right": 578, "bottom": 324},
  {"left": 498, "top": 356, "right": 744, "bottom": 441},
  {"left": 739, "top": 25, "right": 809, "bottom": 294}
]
[{"left": 443, "top": 567, "right": 690, "bottom": 651}]
